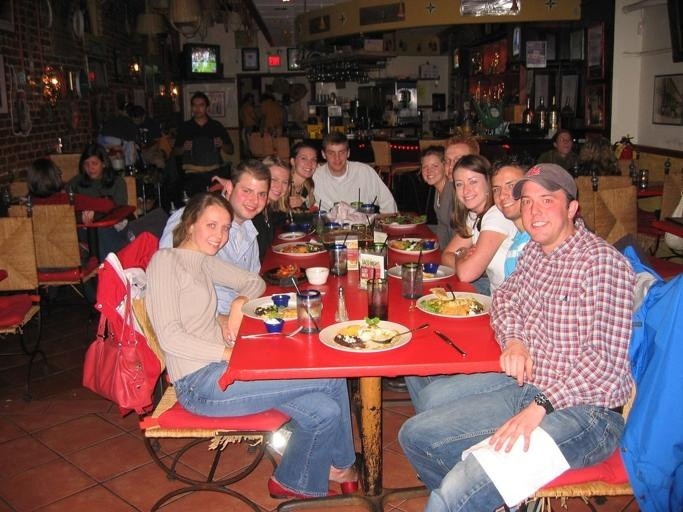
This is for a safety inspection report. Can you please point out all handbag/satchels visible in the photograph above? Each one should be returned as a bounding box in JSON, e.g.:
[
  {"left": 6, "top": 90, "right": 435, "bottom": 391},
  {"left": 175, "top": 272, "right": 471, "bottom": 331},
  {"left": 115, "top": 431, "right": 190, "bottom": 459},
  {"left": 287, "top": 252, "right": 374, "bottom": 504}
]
[{"left": 80, "top": 273, "right": 162, "bottom": 411}]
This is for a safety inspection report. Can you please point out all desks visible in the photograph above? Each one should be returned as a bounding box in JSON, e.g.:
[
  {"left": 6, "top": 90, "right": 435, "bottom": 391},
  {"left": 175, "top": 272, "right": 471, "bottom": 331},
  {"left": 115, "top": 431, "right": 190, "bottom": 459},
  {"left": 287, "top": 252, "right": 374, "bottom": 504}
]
[{"left": 222, "top": 225, "right": 503, "bottom": 510}]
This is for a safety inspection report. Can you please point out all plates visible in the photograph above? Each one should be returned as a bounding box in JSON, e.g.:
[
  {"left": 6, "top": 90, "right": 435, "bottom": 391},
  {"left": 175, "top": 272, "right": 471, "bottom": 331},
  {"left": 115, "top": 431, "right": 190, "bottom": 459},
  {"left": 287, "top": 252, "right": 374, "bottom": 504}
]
[
  {"left": 415, "top": 292, "right": 492, "bottom": 319},
  {"left": 318, "top": 318, "right": 414, "bottom": 354},
  {"left": 240, "top": 292, "right": 322, "bottom": 323},
  {"left": 260, "top": 267, "right": 307, "bottom": 287},
  {"left": 277, "top": 231, "right": 303, "bottom": 240},
  {"left": 386, "top": 238, "right": 439, "bottom": 255},
  {"left": 387, "top": 261, "right": 454, "bottom": 282},
  {"left": 272, "top": 241, "right": 328, "bottom": 257},
  {"left": 381, "top": 223, "right": 417, "bottom": 229}
]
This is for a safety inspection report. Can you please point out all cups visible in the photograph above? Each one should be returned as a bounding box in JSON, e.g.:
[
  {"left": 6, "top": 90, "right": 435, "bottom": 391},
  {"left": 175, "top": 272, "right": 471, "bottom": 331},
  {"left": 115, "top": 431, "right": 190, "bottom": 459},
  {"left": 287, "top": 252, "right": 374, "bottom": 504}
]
[
  {"left": 366, "top": 278, "right": 387, "bottom": 320},
  {"left": 304, "top": 267, "right": 330, "bottom": 285},
  {"left": 400, "top": 262, "right": 422, "bottom": 297},
  {"left": 271, "top": 296, "right": 289, "bottom": 306},
  {"left": 329, "top": 246, "right": 348, "bottom": 275},
  {"left": 263, "top": 318, "right": 285, "bottom": 332},
  {"left": 318, "top": 202, "right": 375, "bottom": 246},
  {"left": 370, "top": 242, "right": 388, "bottom": 269}
]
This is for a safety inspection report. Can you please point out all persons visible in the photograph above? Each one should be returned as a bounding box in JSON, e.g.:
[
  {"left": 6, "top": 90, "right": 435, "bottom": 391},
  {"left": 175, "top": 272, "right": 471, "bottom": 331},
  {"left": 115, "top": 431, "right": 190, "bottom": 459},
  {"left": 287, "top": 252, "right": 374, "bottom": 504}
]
[
  {"left": 241, "top": 94, "right": 282, "bottom": 133},
  {"left": 397, "top": 163, "right": 635, "bottom": 510},
  {"left": 172, "top": 93, "right": 233, "bottom": 186},
  {"left": 26, "top": 105, "right": 157, "bottom": 266},
  {"left": 405, "top": 132, "right": 557, "bottom": 414},
  {"left": 538, "top": 127, "right": 620, "bottom": 177},
  {"left": 146, "top": 190, "right": 359, "bottom": 498},
  {"left": 161, "top": 134, "right": 397, "bottom": 346}
]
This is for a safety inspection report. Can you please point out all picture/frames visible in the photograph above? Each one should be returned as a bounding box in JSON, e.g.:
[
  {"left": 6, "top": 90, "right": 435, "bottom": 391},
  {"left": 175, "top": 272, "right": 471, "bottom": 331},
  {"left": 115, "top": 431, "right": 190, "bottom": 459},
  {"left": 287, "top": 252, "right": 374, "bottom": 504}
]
[
  {"left": 240, "top": 45, "right": 260, "bottom": 72},
  {"left": 285, "top": 46, "right": 304, "bottom": 71},
  {"left": 521, "top": 22, "right": 610, "bottom": 132},
  {"left": 650, "top": 70, "right": 683, "bottom": 129}
]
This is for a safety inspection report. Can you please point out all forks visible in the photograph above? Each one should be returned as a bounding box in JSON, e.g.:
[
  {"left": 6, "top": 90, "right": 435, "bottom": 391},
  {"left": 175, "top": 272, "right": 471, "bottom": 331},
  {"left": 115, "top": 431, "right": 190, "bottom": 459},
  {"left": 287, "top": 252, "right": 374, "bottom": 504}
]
[{"left": 371, "top": 322, "right": 429, "bottom": 344}]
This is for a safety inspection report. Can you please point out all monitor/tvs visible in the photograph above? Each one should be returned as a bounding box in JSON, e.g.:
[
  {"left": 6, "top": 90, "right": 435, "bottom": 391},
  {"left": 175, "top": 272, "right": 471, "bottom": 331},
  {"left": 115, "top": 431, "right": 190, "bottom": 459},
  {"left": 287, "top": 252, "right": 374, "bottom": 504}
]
[{"left": 183, "top": 43, "right": 220, "bottom": 79}]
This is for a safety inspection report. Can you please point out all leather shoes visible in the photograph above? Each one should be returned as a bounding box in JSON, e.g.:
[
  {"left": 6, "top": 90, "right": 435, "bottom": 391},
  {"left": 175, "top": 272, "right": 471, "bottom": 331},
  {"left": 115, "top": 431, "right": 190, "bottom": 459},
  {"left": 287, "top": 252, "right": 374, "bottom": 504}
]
[
  {"left": 385, "top": 378, "right": 409, "bottom": 393},
  {"left": 340, "top": 452, "right": 361, "bottom": 495},
  {"left": 268, "top": 475, "right": 339, "bottom": 502}
]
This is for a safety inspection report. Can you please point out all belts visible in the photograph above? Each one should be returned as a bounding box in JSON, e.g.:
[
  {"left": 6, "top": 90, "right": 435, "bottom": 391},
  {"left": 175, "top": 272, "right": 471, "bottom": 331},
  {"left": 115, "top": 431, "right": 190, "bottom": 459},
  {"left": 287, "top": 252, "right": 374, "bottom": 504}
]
[{"left": 606, "top": 401, "right": 627, "bottom": 416}]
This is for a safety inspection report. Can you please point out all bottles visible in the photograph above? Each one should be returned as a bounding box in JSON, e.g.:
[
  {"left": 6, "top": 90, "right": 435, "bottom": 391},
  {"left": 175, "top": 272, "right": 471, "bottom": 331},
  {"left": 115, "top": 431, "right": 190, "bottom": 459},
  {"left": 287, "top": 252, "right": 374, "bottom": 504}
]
[
  {"left": 334, "top": 286, "right": 348, "bottom": 323},
  {"left": 285, "top": 180, "right": 305, "bottom": 227}
]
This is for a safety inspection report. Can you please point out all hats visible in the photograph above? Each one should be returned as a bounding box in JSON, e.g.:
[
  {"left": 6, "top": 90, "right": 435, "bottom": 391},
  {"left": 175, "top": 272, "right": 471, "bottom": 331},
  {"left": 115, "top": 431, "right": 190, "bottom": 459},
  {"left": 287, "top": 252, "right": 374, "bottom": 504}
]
[{"left": 511, "top": 162, "right": 577, "bottom": 201}]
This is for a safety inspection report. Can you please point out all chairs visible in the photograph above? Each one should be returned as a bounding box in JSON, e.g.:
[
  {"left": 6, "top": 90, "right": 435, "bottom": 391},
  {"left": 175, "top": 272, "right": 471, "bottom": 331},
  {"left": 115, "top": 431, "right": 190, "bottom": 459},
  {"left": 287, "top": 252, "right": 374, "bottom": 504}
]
[
  {"left": 516, "top": 280, "right": 681, "bottom": 511},
  {"left": 234, "top": 135, "right": 681, "bottom": 279},
  {"left": 96, "top": 233, "right": 291, "bottom": 509},
  {"left": 1, "top": 133, "right": 230, "bottom": 390}
]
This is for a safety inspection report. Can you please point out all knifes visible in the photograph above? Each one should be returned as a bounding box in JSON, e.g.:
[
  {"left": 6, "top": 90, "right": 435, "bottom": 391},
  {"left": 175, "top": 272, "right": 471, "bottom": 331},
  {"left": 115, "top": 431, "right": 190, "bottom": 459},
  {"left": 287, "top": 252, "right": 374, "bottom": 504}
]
[{"left": 433, "top": 328, "right": 465, "bottom": 357}]
